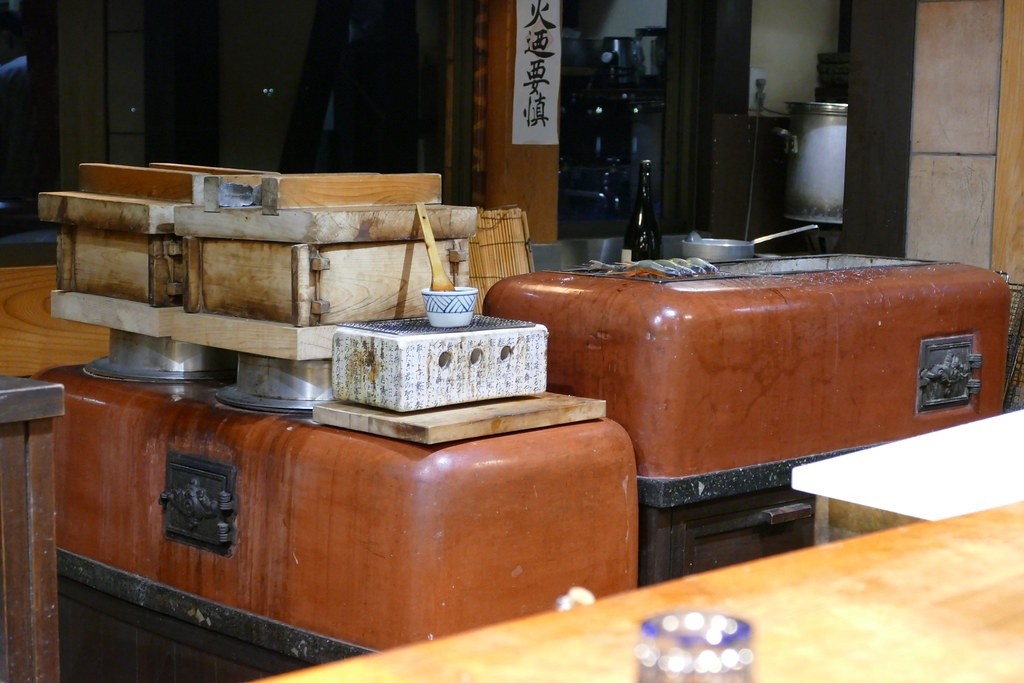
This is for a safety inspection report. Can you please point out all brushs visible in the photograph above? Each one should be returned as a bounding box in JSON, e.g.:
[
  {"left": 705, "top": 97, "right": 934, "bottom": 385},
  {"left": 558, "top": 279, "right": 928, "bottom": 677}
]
[{"left": 416, "top": 202, "right": 453, "bottom": 291}]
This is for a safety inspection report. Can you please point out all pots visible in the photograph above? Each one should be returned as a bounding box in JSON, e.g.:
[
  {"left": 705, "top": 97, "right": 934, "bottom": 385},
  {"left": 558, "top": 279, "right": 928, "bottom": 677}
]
[{"left": 680, "top": 222, "right": 820, "bottom": 262}]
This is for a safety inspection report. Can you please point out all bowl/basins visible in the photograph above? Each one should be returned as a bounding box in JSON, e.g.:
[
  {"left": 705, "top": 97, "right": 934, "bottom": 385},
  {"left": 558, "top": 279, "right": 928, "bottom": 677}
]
[{"left": 422, "top": 286, "right": 478, "bottom": 328}]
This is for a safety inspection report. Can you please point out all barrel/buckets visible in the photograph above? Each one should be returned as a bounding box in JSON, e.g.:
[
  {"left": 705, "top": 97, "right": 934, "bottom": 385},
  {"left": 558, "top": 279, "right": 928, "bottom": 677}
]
[{"left": 773, "top": 99, "right": 848, "bottom": 224}]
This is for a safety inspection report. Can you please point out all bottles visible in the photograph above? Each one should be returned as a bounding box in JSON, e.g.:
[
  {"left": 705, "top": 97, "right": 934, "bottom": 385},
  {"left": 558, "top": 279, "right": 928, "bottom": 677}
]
[{"left": 620, "top": 159, "right": 662, "bottom": 263}]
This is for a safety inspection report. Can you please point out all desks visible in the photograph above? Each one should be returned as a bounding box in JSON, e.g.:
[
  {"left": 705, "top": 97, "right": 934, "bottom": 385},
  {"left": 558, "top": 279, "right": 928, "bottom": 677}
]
[
  {"left": 253, "top": 502, "right": 1023, "bottom": 683},
  {"left": 793, "top": 409, "right": 1022, "bottom": 523}
]
